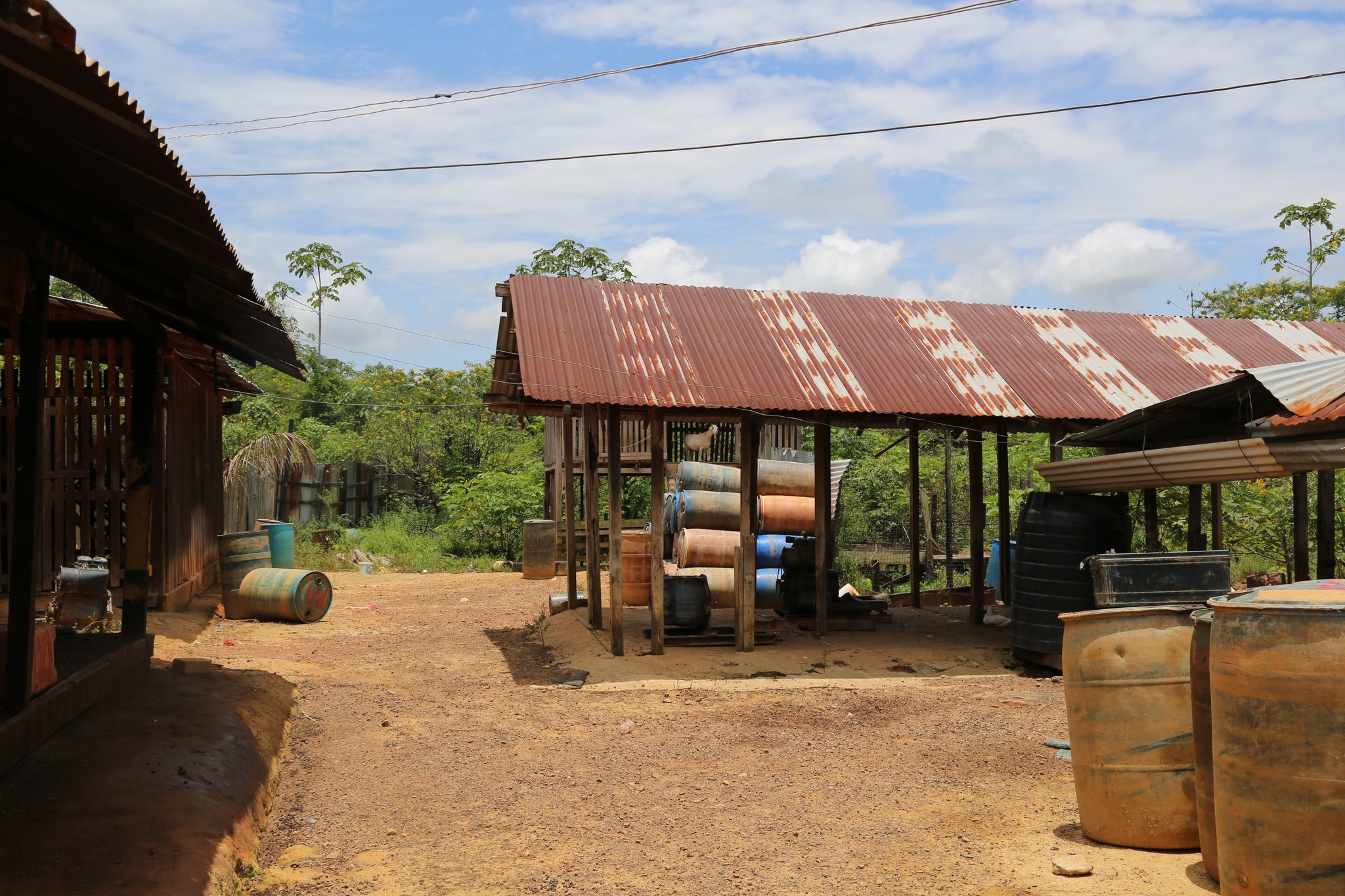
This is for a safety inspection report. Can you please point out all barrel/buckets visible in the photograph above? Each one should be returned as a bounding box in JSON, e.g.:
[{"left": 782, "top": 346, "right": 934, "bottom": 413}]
[
  {"left": 344, "top": 528, "right": 360, "bottom": 539},
  {"left": 490, "top": 561, "right": 523, "bottom": 573},
  {"left": 948, "top": 585, "right": 996, "bottom": 606},
  {"left": 358, "top": 562, "right": 373, "bottom": 576},
  {"left": 986, "top": 538, "right": 1017, "bottom": 601},
  {"left": 260, "top": 523, "right": 295, "bottom": 569},
  {"left": 554, "top": 561, "right": 567, "bottom": 576},
  {"left": 522, "top": 519, "right": 556, "bottom": 579},
  {"left": 254, "top": 518, "right": 285, "bottom": 531},
  {"left": 1058, "top": 580, "right": 1344, "bottom": 896},
  {"left": 621, "top": 458, "right": 814, "bottom": 609},
  {"left": 238, "top": 568, "right": 333, "bottom": 624},
  {"left": 311, "top": 530, "right": 334, "bottom": 550},
  {"left": 217, "top": 530, "right": 272, "bottom": 620}
]
